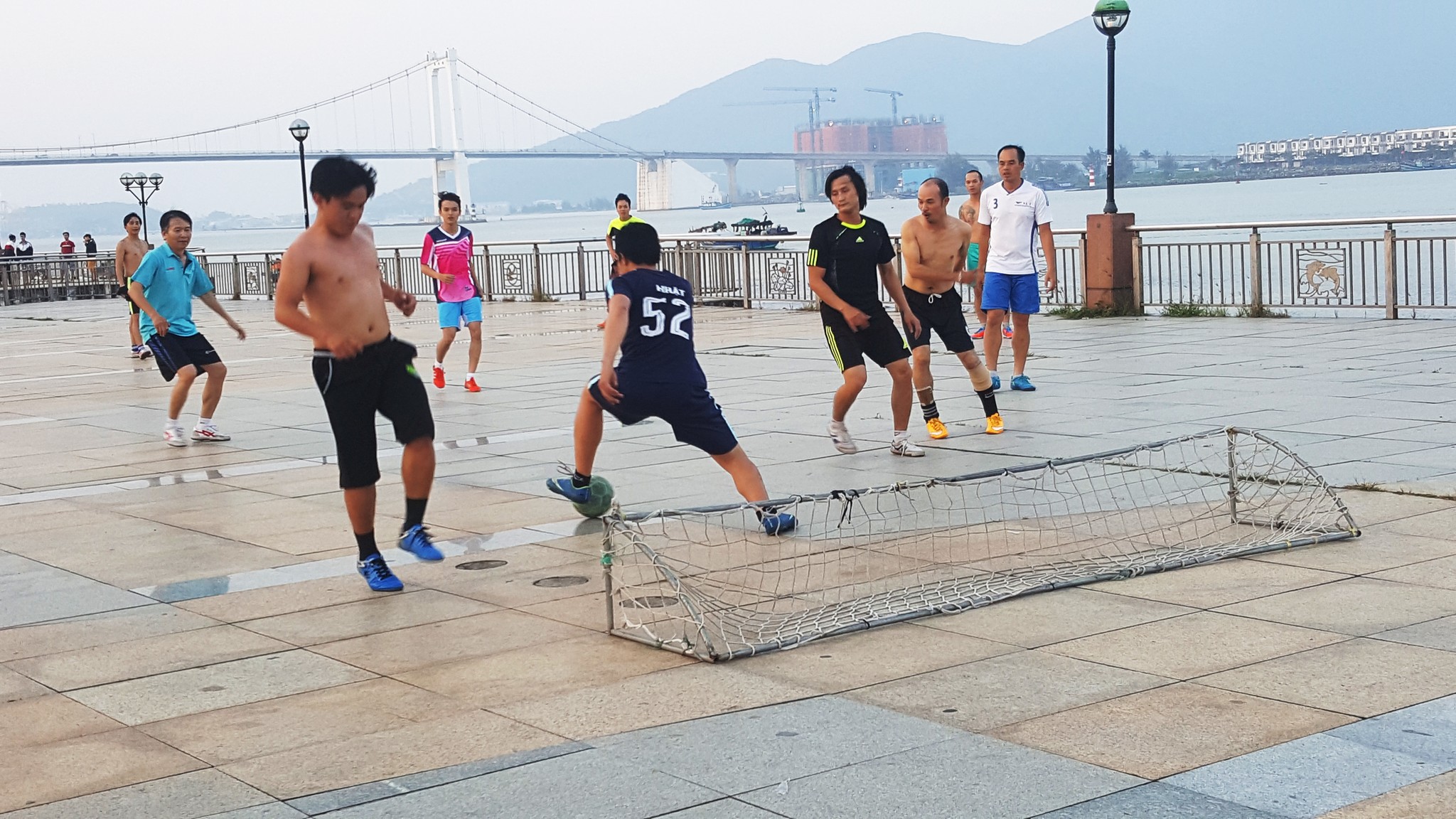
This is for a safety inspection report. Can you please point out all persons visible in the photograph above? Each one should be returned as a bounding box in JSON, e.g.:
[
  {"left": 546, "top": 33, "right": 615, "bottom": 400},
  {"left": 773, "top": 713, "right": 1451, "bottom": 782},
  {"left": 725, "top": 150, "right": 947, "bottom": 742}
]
[
  {"left": 546, "top": 223, "right": 801, "bottom": 535},
  {"left": 128, "top": 210, "right": 245, "bottom": 447},
  {"left": 420, "top": 191, "right": 486, "bottom": 392},
  {"left": 806, "top": 146, "right": 1056, "bottom": 458},
  {"left": 599, "top": 193, "right": 647, "bottom": 331},
  {"left": 275, "top": 156, "right": 446, "bottom": 591},
  {"left": 0, "top": 212, "right": 150, "bottom": 360}
]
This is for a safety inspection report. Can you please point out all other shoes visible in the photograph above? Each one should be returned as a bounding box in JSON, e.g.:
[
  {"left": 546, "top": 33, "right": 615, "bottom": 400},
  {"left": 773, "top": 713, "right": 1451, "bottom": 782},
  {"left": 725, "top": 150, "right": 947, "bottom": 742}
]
[{"left": 597, "top": 321, "right": 606, "bottom": 328}]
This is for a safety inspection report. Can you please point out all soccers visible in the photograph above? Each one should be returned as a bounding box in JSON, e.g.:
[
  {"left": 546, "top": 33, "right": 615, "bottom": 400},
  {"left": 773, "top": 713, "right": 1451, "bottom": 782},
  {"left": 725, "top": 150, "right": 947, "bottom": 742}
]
[{"left": 573, "top": 475, "right": 613, "bottom": 521}]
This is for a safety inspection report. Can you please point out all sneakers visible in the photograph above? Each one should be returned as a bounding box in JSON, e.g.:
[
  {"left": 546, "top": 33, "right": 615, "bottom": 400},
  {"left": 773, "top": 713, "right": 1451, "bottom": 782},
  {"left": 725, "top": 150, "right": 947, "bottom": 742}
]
[
  {"left": 925, "top": 418, "right": 948, "bottom": 438},
  {"left": 985, "top": 413, "right": 1004, "bottom": 434},
  {"left": 138, "top": 349, "right": 151, "bottom": 359},
  {"left": 1003, "top": 327, "right": 1014, "bottom": 339},
  {"left": 433, "top": 365, "right": 445, "bottom": 388},
  {"left": 163, "top": 425, "right": 187, "bottom": 446},
  {"left": 992, "top": 376, "right": 1001, "bottom": 390},
  {"left": 547, "top": 460, "right": 591, "bottom": 504},
  {"left": 191, "top": 425, "right": 231, "bottom": 441},
  {"left": 464, "top": 377, "right": 481, "bottom": 392},
  {"left": 356, "top": 553, "right": 403, "bottom": 592},
  {"left": 397, "top": 522, "right": 444, "bottom": 561},
  {"left": 1010, "top": 374, "right": 1036, "bottom": 391},
  {"left": 971, "top": 327, "right": 985, "bottom": 339},
  {"left": 890, "top": 435, "right": 925, "bottom": 457},
  {"left": 762, "top": 513, "right": 798, "bottom": 536},
  {"left": 131, "top": 349, "right": 155, "bottom": 358},
  {"left": 827, "top": 421, "right": 857, "bottom": 454}
]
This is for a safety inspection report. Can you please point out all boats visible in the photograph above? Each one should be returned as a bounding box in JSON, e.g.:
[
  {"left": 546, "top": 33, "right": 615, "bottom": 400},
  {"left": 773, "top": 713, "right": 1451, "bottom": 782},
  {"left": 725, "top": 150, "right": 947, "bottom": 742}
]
[
  {"left": 698, "top": 192, "right": 732, "bottom": 210},
  {"left": 796, "top": 192, "right": 805, "bottom": 213},
  {"left": 685, "top": 206, "right": 798, "bottom": 249},
  {"left": 897, "top": 190, "right": 919, "bottom": 200},
  {"left": 1400, "top": 157, "right": 1456, "bottom": 172},
  {"left": 1032, "top": 176, "right": 1064, "bottom": 190}
]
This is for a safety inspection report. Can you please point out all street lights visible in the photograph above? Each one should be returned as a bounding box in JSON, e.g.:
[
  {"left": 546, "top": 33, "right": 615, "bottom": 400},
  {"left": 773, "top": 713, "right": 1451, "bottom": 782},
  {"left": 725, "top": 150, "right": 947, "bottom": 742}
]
[
  {"left": 288, "top": 118, "right": 311, "bottom": 228},
  {"left": 119, "top": 172, "right": 163, "bottom": 249},
  {"left": 1085, "top": 0, "right": 1137, "bottom": 313}
]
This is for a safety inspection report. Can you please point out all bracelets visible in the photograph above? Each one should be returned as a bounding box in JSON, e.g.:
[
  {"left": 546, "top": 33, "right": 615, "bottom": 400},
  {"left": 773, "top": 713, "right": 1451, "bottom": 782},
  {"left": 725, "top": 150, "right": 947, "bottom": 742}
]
[{"left": 959, "top": 272, "right": 962, "bottom": 283}]
